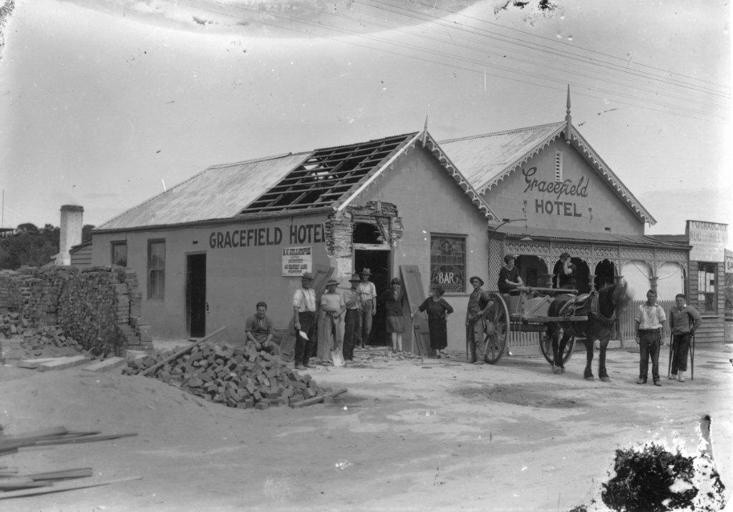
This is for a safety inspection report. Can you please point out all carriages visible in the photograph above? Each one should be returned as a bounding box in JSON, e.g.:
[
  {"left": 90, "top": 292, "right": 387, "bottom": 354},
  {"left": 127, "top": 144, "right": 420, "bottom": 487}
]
[{"left": 466, "top": 257, "right": 632, "bottom": 386}]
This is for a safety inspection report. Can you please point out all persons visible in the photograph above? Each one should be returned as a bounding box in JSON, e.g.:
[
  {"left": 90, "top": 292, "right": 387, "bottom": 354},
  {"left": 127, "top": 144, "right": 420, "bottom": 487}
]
[
  {"left": 292, "top": 267, "right": 378, "bottom": 371},
  {"left": 499, "top": 255, "right": 524, "bottom": 297},
  {"left": 410, "top": 284, "right": 453, "bottom": 359},
  {"left": 668, "top": 293, "right": 702, "bottom": 382},
  {"left": 244, "top": 302, "right": 281, "bottom": 355},
  {"left": 632, "top": 289, "right": 666, "bottom": 385},
  {"left": 552, "top": 252, "right": 577, "bottom": 288},
  {"left": 380, "top": 278, "right": 405, "bottom": 354},
  {"left": 465, "top": 276, "right": 493, "bottom": 364}
]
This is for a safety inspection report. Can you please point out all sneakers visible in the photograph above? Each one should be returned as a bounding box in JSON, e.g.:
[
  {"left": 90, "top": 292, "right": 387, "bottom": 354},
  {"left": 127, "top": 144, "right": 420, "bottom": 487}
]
[
  {"left": 295, "top": 363, "right": 306, "bottom": 370},
  {"left": 668, "top": 374, "right": 675, "bottom": 379},
  {"left": 679, "top": 374, "right": 684, "bottom": 382},
  {"left": 637, "top": 377, "right": 647, "bottom": 384},
  {"left": 654, "top": 378, "right": 661, "bottom": 385},
  {"left": 464, "top": 357, "right": 486, "bottom": 364},
  {"left": 304, "top": 363, "right": 316, "bottom": 368}
]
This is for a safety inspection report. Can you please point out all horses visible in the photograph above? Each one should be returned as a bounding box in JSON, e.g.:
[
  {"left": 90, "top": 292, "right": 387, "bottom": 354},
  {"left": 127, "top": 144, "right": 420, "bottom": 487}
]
[{"left": 541, "top": 281, "right": 628, "bottom": 383}]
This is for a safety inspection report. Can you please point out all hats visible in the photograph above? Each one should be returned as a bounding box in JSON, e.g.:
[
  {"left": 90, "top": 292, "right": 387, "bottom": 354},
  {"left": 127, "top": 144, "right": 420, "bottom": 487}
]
[
  {"left": 359, "top": 267, "right": 373, "bottom": 275},
  {"left": 326, "top": 280, "right": 340, "bottom": 287},
  {"left": 470, "top": 276, "right": 484, "bottom": 286},
  {"left": 349, "top": 274, "right": 363, "bottom": 282},
  {"left": 302, "top": 272, "right": 314, "bottom": 280}
]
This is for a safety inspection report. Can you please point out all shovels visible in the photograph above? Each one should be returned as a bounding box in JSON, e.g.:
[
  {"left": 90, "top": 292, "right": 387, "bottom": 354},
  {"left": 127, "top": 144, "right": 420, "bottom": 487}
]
[
  {"left": 295, "top": 324, "right": 310, "bottom": 341},
  {"left": 327, "top": 310, "right": 346, "bottom": 367}
]
